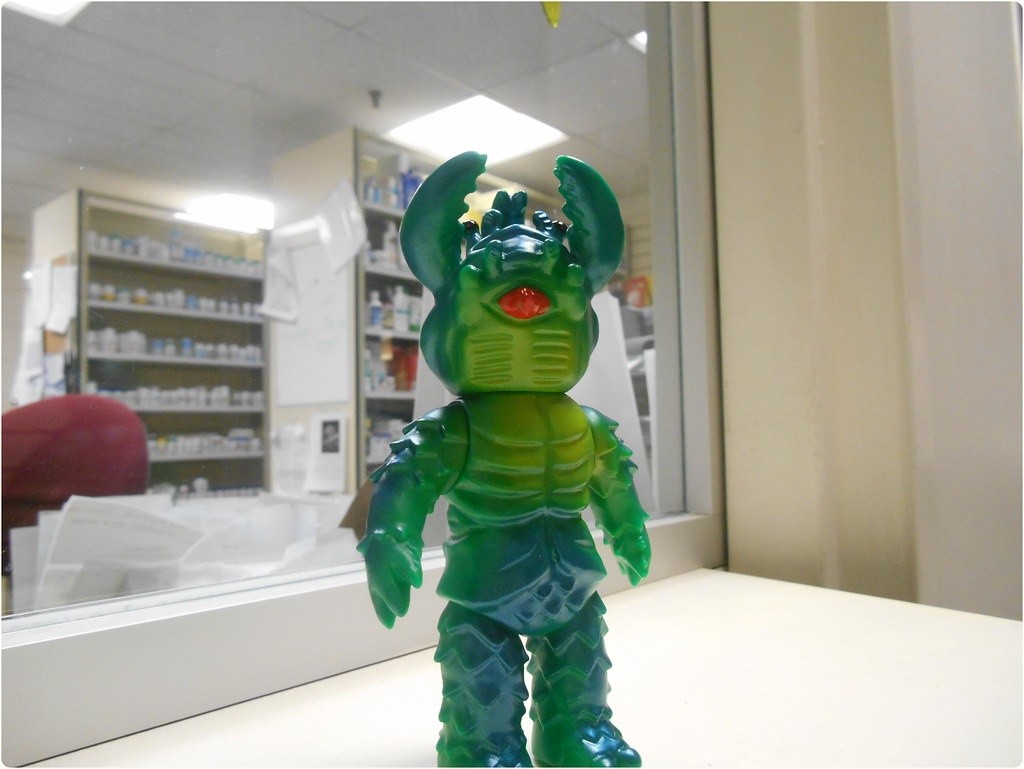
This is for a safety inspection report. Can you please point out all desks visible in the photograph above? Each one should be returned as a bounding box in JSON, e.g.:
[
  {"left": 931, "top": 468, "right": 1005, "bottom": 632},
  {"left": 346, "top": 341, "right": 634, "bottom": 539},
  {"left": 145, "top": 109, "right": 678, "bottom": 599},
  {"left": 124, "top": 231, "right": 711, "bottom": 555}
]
[{"left": 23, "top": 567, "right": 1024, "bottom": 769}]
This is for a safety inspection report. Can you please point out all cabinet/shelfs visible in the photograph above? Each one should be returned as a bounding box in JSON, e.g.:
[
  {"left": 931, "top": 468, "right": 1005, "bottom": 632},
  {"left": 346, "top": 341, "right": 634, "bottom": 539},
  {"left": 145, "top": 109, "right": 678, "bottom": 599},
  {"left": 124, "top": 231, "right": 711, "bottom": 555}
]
[
  {"left": 30, "top": 184, "right": 270, "bottom": 493},
  {"left": 266, "top": 122, "right": 633, "bottom": 498}
]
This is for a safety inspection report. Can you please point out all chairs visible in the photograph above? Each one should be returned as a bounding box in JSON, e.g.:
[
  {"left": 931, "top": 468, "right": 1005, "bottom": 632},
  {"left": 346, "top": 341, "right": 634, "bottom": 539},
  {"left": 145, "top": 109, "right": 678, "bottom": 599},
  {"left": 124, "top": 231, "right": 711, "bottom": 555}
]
[{"left": 2, "top": 393, "right": 148, "bottom": 577}]
